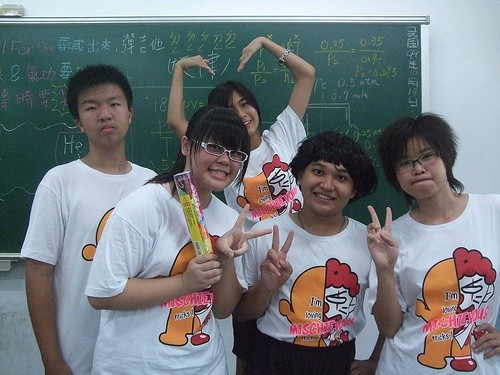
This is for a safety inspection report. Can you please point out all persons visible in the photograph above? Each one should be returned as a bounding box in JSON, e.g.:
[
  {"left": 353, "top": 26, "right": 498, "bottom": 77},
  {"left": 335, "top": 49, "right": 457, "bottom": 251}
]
[
  {"left": 20, "top": 64, "right": 157, "bottom": 375},
  {"left": 166, "top": 37, "right": 317, "bottom": 232},
  {"left": 84, "top": 105, "right": 273, "bottom": 375},
  {"left": 366, "top": 112, "right": 500, "bottom": 374},
  {"left": 231, "top": 131, "right": 386, "bottom": 375}
]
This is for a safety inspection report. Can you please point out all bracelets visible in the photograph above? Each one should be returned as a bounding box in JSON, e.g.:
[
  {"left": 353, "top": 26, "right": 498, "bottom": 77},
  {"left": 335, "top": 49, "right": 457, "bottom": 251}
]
[{"left": 277, "top": 49, "right": 293, "bottom": 66}]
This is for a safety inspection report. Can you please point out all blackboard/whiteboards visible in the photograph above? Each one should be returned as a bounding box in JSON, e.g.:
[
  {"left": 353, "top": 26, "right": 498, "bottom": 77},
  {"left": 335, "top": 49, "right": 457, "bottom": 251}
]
[{"left": 1, "top": 13, "right": 432, "bottom": 259}]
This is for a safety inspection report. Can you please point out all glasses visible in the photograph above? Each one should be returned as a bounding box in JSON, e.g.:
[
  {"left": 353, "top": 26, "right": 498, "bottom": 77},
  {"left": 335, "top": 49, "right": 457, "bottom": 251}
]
[
  {"left": 190, "top": 138, "right": 248, "bottom": 162},
  {"left": 394, "top": 150, "right": 441, "bottom": 171}
]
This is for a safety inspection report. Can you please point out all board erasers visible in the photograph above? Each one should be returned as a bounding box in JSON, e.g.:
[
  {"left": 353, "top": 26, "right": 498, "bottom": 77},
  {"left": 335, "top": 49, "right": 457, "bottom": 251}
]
[{"left": 1, "top": 4, "right": 24, "bottom": 17}]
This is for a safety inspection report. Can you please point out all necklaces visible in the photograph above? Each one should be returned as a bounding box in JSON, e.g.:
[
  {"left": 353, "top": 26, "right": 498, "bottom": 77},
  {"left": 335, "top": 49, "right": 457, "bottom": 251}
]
[{"left": 298, "top": 210, "right": 346, "bottom": 233}]
